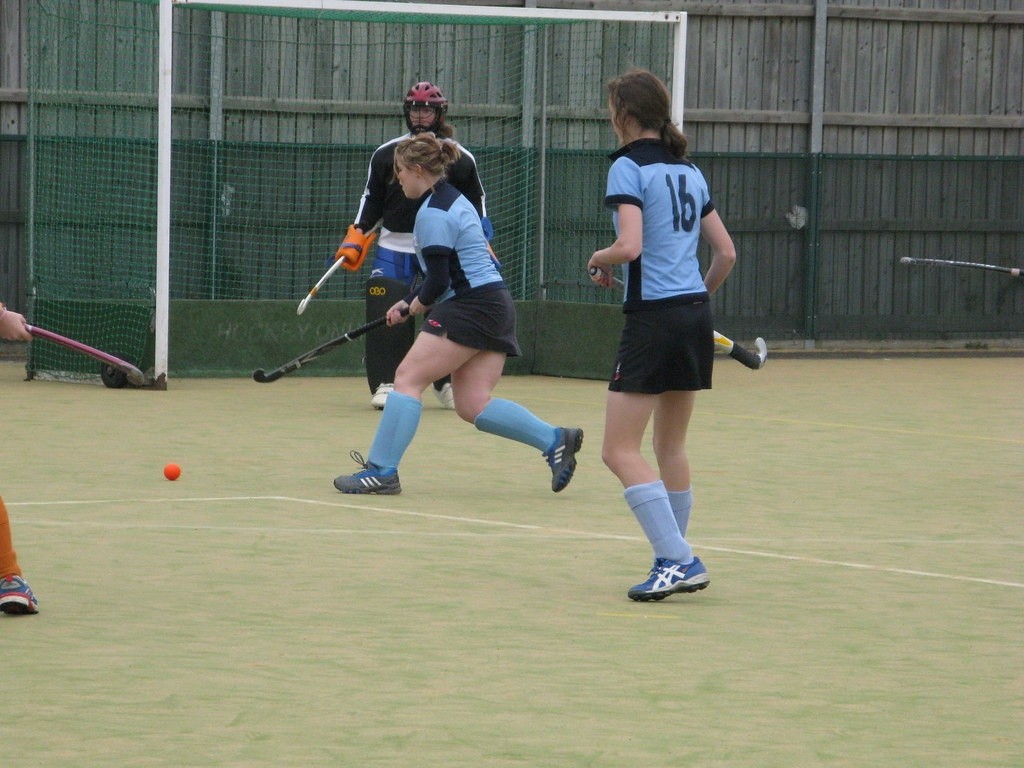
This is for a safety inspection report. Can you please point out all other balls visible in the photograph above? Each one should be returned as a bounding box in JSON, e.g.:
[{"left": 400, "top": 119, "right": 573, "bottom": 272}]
[{"left": 164, "top": 463, "right": 181, "bottom": 480}]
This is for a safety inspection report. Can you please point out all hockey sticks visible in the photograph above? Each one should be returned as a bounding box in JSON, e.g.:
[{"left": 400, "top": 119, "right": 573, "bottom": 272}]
[
  {"left": 590, "top": 266, "right": 767, "bottom": 370},
  {"left": 296, "top": 224, "right": 378, "bottom": 316},
  {"left": 900, "top": 256, "right": 1024, "bottom": 277},
  {"left": 24, "top": 322, "right": 145, "bottom": 386},
  {"left": 253, "top": 306, "right": 410, "bottom": 383}
]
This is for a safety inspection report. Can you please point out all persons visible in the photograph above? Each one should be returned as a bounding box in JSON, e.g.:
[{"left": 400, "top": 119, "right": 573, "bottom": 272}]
[
  {"left": 339, "top": 81, "right": 493, "bottom": 411},
  {"left": 587, "top": 69, "right": 737, "bottom": 602},
  {"left": 0, "top": 302, "right": 41, "bottom": 614},
  {"left": 333, "top": 134, "right": 584, "bottom": 496}
]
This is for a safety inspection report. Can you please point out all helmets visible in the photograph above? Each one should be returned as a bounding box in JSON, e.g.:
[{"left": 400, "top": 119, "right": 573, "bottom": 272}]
[{"left": 403, "top": 82, "right": 447, "bottom": 107}]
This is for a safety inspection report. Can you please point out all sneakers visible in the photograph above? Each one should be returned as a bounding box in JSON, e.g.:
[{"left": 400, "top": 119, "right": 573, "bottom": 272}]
[
  {"left": 336, "top": 452, "right": 402, "bottom": 496},
  {"left": 544, "top": 426, "right": 587, "bottom": 492},
  {"left": 627, "top": 556, "right": 709, "bottom": 603},
  {"left": 0, "top": 575, "right": 40, "bottom": 617}
]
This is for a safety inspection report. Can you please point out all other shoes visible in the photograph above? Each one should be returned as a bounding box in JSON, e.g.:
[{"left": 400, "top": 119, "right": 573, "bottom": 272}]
[
  {"left": 370, "top": 381, "right": 396, "bottom": 409},
  {"left": 433, "top": 383, "right": 457, "bottom": 410}
]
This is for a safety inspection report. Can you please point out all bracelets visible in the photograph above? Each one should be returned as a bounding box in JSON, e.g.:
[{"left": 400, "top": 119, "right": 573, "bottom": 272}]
[{"left": 0, "top": 306, "right": 7, "bottom": 320}]
